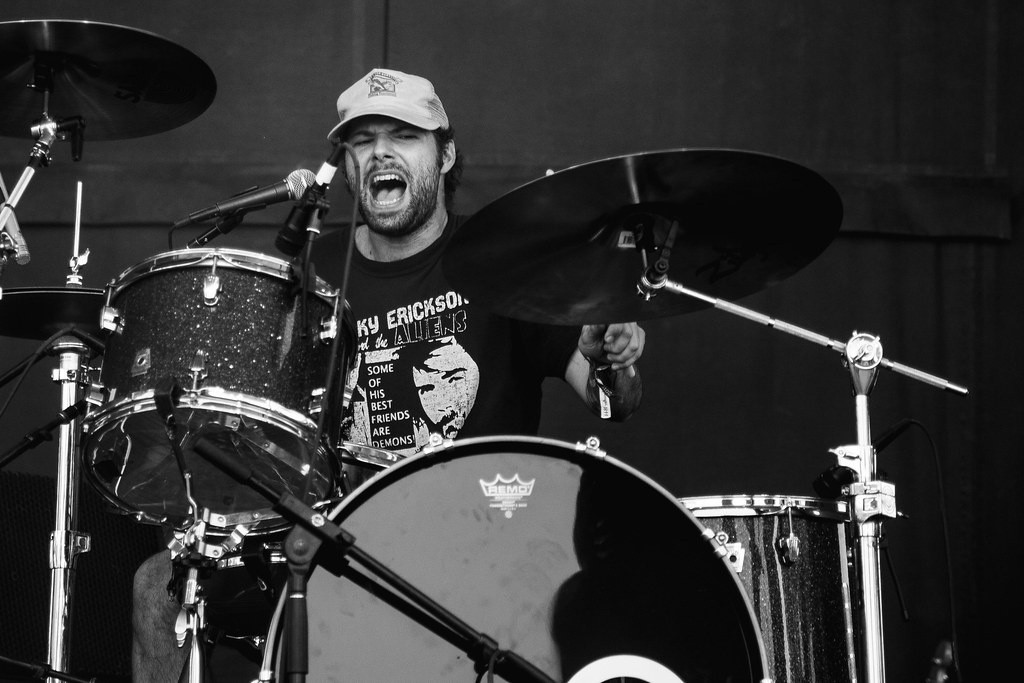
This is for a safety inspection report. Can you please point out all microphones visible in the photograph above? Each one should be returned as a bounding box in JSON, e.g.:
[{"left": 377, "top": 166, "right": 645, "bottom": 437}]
[
  {"left": 174, "top": 169, "right": 317, "bottom": 229},
  {"left": 0, "top": 174, "right": 31, "bottom": 265},
  {"left": 813, "top": 416, "right": 912, "bottom": 502},
  {"left": 275, "top": 143, "right": 348, "bottom": 257}
]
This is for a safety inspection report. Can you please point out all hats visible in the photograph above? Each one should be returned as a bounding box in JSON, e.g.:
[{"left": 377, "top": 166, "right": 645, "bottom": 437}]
[{"left": 327, "top": 68, "right": 449, "bottom": 147}]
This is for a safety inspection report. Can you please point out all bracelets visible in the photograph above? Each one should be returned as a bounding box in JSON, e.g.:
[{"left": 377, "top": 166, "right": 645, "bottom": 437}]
[{"left": 581, "top": 353, "right": 622, "bottom": 419}]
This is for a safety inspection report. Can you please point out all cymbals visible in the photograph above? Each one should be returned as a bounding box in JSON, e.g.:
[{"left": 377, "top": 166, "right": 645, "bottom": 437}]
[
  {"left": 443, "top": 146, "right": 846, "bottom": 324},
  {"left": 0, "top": 17, "right": 218, "bottom": 146},
  {"left": 1, "top": 285, "right": 111, "bottom": 345}
]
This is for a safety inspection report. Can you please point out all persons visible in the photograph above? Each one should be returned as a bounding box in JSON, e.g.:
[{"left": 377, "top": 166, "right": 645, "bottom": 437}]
[{"left": 130, "top": 65, "right": 647, "bottom": 683}]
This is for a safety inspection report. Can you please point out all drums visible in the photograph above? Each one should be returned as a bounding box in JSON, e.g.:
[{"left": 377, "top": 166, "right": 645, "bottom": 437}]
[
  {"left": 79, "top": 247, "right": 355, "bottom": 537},
  {"left": 252, "top": 434, "right": 880, "bottom": 683}
]
